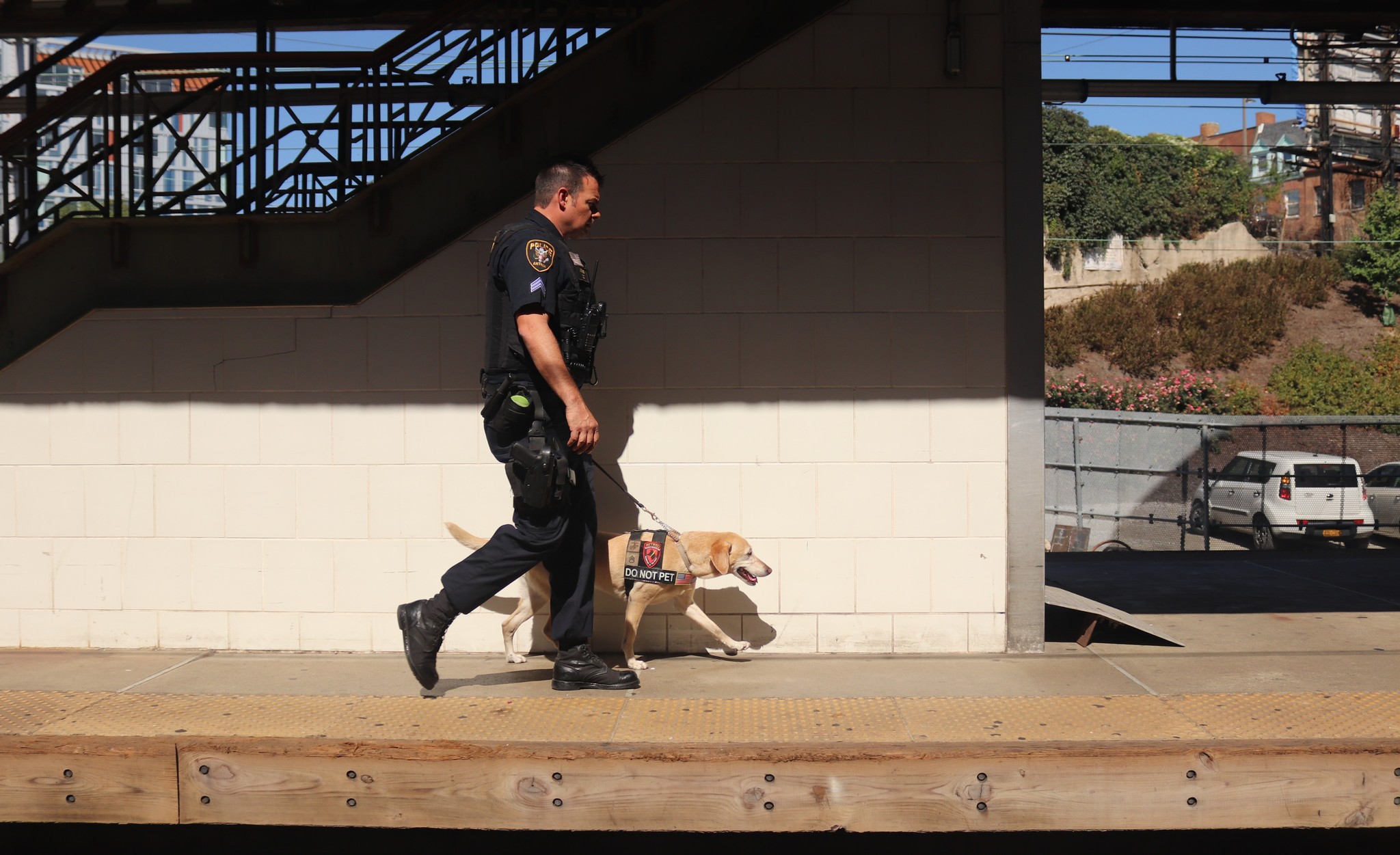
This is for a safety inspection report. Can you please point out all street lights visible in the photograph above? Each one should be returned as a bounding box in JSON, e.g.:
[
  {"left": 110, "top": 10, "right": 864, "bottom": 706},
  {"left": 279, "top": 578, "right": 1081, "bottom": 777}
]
[{"left": 1242, "top": 99, "right": 1255, "bottom": 166}]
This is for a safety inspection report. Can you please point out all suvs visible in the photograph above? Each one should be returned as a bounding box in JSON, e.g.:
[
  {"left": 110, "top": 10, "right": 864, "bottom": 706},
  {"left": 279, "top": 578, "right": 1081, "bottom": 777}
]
[{"left": 1187, "top": 450, "right": 1375, "bottom": 552}]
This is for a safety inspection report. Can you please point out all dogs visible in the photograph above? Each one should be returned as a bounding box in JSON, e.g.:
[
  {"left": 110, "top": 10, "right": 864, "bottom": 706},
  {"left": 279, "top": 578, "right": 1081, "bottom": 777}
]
[{"left": 444, "top": 522, "right": 772, "bottom": 670}]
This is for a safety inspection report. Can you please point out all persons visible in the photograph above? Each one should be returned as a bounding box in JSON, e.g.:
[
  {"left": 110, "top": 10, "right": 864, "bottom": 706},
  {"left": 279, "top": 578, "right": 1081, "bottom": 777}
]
[{"left": 396, "top": 160, "right": 641, "bottom": 691}]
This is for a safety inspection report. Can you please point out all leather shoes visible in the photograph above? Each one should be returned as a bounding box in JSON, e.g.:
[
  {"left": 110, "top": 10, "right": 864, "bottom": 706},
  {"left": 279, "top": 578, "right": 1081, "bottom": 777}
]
[
  {"left": 398, "top": 599, "right": 448, "bottom": 691},
  {"left": 551, "top": 643, "right": 639, "bottom": 691}
]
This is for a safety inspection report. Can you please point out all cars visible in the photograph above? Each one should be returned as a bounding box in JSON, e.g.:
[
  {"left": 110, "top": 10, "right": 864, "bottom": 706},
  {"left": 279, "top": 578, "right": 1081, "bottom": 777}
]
[{"left": 1364, "top": 462, "right": 1400, "bottom": 526}]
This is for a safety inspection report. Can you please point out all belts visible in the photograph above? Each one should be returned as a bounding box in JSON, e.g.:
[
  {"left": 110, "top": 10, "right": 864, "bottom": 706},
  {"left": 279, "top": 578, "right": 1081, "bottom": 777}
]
[{"left": 496, "top": 383, "right": 543, "bottom": 393}]
